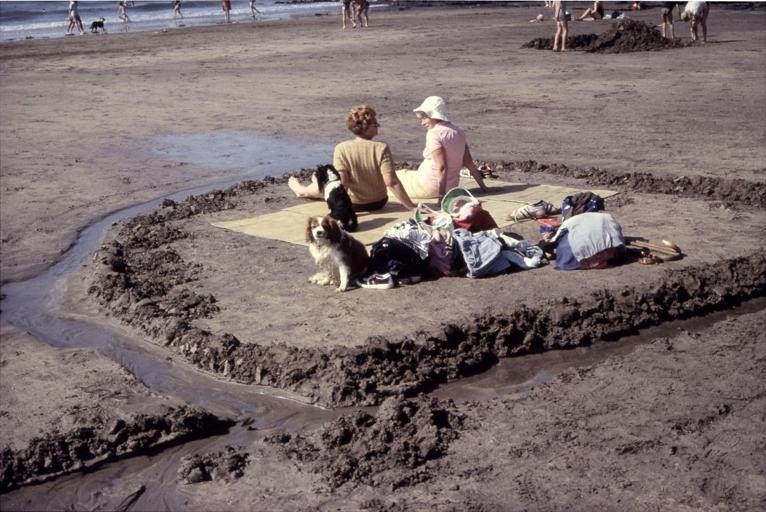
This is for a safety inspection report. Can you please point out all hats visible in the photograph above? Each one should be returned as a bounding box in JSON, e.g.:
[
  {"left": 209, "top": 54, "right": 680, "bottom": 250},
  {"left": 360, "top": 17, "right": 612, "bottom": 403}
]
[{"left": 411, "top": 97, "right": 450, "bottom": 124}]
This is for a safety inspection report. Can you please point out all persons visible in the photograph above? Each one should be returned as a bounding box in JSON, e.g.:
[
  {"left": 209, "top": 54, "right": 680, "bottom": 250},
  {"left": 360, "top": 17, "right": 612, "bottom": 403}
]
[
  {"left": 286, "top": 103, "right": 431, "bottom": 214},
  {"left": 221, "top": 0, "right": 232, "bottom": 24},
  {"left": 385, "top": 94, "right": 504, "bottom": 209},
  {"left": 680, "top": 1, "right": 710, "bottom": 43},
  {"left": 69, "top": 1, "right": 87, "bottom": 35},
  {"left": 66, "top": 1, "right": 76, "bottom": 35},
  {"left": 248, "top": 0, "right": 261, "bottom": 18},
  {"left": 172, "top": 0, "right": 184, "bottom": 20},
  {"left": 116, "top": 0, "right": 136, "bottom": 23},
  {"left": 661, "top": 1, "right": 680, "bottom": 41},
  {"left": 552, "top": 0, "right": 569, "bottom": 52},
  {"left": 340, "top": 0, "right": 370, "bottom": 30},
  {"left": 545, "top": 1, "right": 642, "bottom": 23}
]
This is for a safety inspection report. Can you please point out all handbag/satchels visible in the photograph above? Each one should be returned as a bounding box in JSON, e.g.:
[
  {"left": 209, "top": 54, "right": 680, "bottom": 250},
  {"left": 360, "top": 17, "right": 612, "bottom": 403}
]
[
  {"left": 414, "top": 201, "right": 454, "bottom": 232},
  {"left": 438, "top": 187, "right": 499, "bottom": 232}
]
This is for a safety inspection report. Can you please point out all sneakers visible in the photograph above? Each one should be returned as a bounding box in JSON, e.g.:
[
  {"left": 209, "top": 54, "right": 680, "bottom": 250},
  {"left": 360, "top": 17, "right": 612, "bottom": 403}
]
[
  {"left": 379, "top": 259, "right": 424, "bottom": 287},
  {"left": 528, "top": 200, "right": 562, "bottom": 214},
  {"left": 354, "top": 271, "right": 397, "bottom": 290},
  {"left": 509, "top": 205, "right": 546, "bottom": 219}
]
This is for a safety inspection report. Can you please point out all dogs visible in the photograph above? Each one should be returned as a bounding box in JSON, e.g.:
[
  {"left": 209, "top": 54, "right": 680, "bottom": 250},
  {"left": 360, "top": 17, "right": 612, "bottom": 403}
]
[
  {"left": 314, "top": 163, "right": 358, "bottom": 232},
  {"left": 89, "top": 17, "right": 106, "bottom": 33},
  {"left": 304, "top": 214, "right": 370, "bottom": 293}
]
